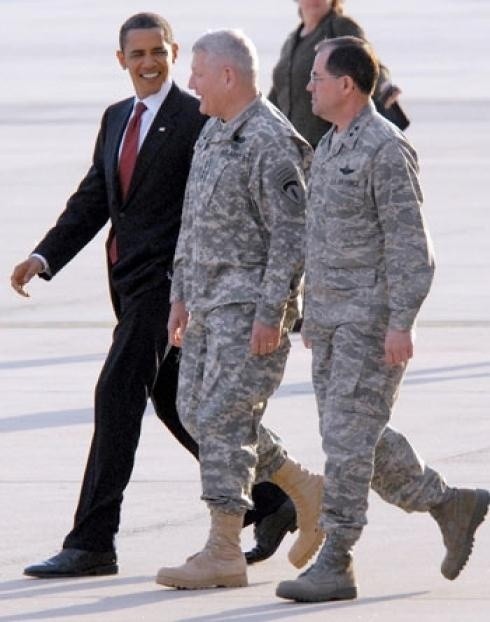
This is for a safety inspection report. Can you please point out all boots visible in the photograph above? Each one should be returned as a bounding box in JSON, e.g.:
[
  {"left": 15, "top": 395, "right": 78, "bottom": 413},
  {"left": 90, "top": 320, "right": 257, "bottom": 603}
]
[
  {"left": 270, "top": 456, "right": 325, "bottom": 567},
  {"left": 159, "top": 512, "right": 248, "bottom": 590},
  {"left": 275, "top": 552, "right": 358, "bottom": 603},
  {"left": 431, "top": 487, "right": 489, "bottom": 583}
]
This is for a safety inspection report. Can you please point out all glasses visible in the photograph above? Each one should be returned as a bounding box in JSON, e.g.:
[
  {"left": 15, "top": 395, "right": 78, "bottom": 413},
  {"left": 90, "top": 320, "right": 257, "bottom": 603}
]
[{"left": 310, "top": 73, "right": 338, "bottom": 83}]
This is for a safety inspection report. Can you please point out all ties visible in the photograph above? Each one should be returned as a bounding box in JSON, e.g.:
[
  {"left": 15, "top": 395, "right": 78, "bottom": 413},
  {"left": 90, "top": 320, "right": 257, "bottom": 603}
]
[{"left": 106, "top": 102, "right": 144, "bottom": 264}]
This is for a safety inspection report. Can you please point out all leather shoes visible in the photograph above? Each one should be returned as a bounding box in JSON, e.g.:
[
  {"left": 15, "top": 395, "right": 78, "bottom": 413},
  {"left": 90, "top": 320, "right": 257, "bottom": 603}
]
[
  {"left": 248, "top": 504, "right": 296, "bottom": 564},
  {"left": 23, "top": 546, "right": 118, "bottom": 579}
]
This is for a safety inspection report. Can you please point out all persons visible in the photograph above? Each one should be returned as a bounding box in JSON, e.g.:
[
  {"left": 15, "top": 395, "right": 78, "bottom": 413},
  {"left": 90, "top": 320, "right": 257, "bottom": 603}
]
[
  {"left": 275, "top": 34, "right": 490, "bottom": 601},
  {"left": 151, "top": 29, "right": 329, "bottom": 590},
  {"left": 265, "top": 0, "right": 412, "bottom": 334},
  {"left": 10, "top": 11, "right": 301, "bottom": 578}
]
